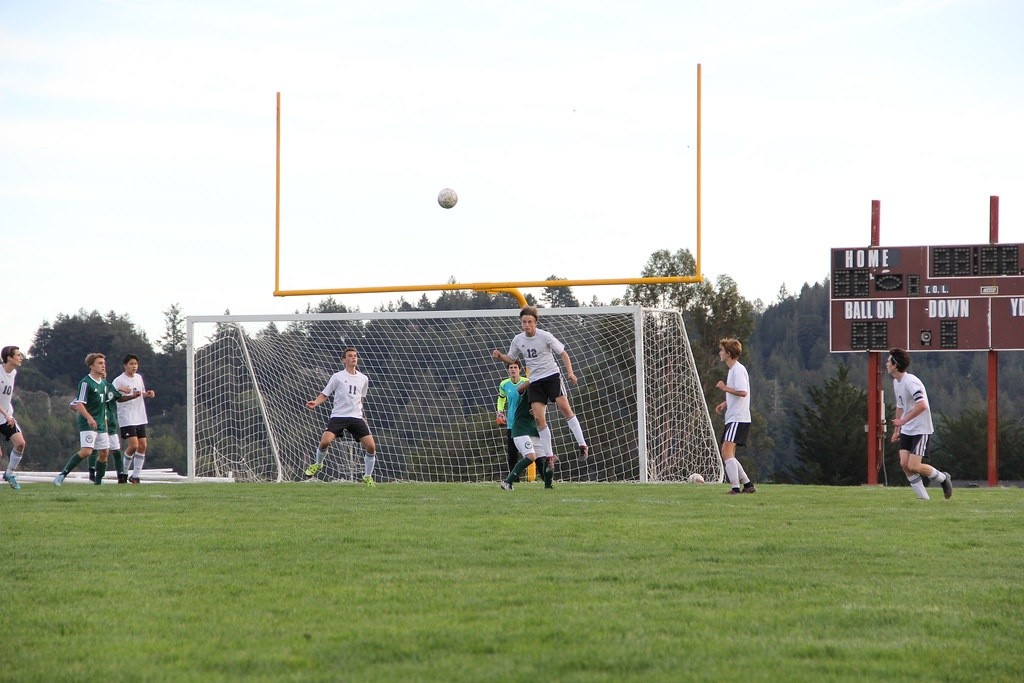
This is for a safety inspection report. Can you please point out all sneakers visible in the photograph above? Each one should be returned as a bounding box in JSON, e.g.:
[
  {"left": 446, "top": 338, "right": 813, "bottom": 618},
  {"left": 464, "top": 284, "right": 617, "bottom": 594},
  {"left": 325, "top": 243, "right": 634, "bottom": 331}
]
[
  {"left": 3, "top": 472, "right": 21, "bottom": 489},
  {"left": 305, "top": 463, "right": 324, "bottom": 476},
  {"left": 363, "top": 475, "right": 375, "bottom": 487},
  {"left": 578, "top": 444, "right": 589, "bottom": 462},
  {"left": 54, "top": 474, "right": 65, "bottom": 487},
  {"left": 546, "top": 455, "right": 555, "bottom": 472},
  {"left": 940, "top": 472, "right": 952, "bottom": 499},
  {"left": 501, "top": 480, "right": 515, "bottom": 490}
]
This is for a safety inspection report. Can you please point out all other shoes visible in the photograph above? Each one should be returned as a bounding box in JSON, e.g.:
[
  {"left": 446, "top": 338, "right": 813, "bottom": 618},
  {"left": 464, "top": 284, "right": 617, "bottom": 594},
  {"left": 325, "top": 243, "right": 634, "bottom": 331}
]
[
  {"left": 123, "top": 474, "right": 128, "bottom": 483},
  {"left": 741, "top": 487, "right": 756, "bottom": 493},
  {"left": 728, "top": 490, "right": 740, "bottom": 494},
  {"left": 128, "top": 476, "right": 140, "bottom": 483},
  {"left": 89, "top": 475, "right": 96, "bottom": 483}
]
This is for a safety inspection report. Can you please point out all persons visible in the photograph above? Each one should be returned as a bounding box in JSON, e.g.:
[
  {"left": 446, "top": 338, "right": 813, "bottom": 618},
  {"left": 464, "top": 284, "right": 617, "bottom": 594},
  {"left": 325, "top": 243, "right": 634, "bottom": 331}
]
[
  {"left": 113, "top": 354, "right": 155, "bottom": 484},
  {"left": 715, "top": 334, "right": 757, "bottom": 495},
  {"left": 304, "top": 348, "right": 376, "bottom": 488},
  {"left": 52, "top": 353, "right": 109, "bottom": 486},
  {"left": 886, "top": 348, "right": 953, "bottom": 502},
  {"left": 496, "top": 361, "right": 546, "bottom": 482},
  {"left": 69, "top": 370, "right": 139, "bottom": 481},
  {"left": 0, "top": 346, "right": 26, "bottom": 490},
  {"left": 501, "top": 381, "right": 547, "bottom": 491},
  {"left": 493, "top": 306, "right": 588, "bottom": 472}
]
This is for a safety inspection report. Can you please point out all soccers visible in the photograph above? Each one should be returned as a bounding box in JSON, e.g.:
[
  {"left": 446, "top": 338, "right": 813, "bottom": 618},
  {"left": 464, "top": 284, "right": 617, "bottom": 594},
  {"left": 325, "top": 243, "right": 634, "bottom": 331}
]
[
  {"left": 438, "top": 188, "right": 457, "bottom": 209},
  {"left": 689, "top": 473, "right": 704, "bottom": 482}
]
